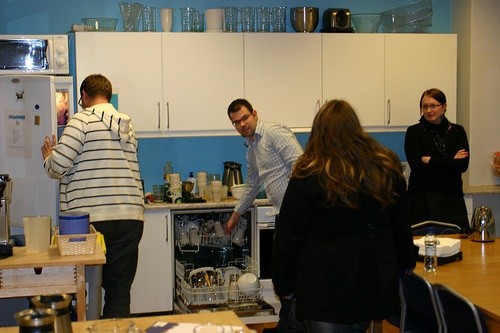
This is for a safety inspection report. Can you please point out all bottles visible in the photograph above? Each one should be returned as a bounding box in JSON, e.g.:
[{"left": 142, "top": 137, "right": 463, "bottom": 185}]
[
  {"left": 163, "top": 161, "right": 197, "bottom": 194},
  {"left": 424, "top": 226, "right": 439, "bottom": 272}
]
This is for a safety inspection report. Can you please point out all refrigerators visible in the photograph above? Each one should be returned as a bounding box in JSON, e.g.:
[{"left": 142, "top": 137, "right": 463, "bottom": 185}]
[{"left": 1, "top": 70, "right": 73, "bottom": 230}]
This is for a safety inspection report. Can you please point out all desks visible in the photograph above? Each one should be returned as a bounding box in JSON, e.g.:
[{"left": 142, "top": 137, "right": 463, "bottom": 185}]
[
  {"left": 412, "top": 233, "right": 500, "bottom": 333},
  {"left": 0, "top": 311, "right": 255, "bottom": 333},
  {"left": 0, "top": 243, "right": 106, "bottom": 322}
]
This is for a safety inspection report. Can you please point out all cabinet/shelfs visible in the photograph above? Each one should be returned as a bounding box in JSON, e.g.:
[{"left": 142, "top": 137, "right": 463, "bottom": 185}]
[
  {"left": 102, "top": 210, "right": 175, "bottom": 314},
  {"left": 68, "top": 32, "right": 457, "bottom": 138}
]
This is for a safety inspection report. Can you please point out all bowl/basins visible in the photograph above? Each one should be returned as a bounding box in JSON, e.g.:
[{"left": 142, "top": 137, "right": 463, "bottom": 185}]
[
  {"left": 237, "top": 272, "right": 260, "bottom": 295},
  {"left": 351, "top": 13, "right": 384, "bottom": 33},
  {"left": 80, "top": 17, "right": 117, "bottom": 32},
  {"left": 230, "top": 183, "right": 247, "bottom": 199},
  {"left": 290, "top": 7, "right": 320, "bottom": 33}
]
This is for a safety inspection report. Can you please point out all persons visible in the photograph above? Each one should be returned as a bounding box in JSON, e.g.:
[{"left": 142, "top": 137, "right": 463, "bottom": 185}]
[
  {"left": 271, "top": 100, "right": 419, "bottom": 333},
  {"left": 41, "top": 75, "right": 146, "bottom": 318},
  {"left": 403, "top": 89, "right": 473, "bottom": 235},
  {"left": 225, "top": 98, "right": 304, "bottom": 234}
]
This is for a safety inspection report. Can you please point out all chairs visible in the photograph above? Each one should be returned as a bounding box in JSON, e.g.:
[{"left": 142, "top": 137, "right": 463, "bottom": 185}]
[{"left": 399, "top": 221, "right": 489, "bottom": 333}]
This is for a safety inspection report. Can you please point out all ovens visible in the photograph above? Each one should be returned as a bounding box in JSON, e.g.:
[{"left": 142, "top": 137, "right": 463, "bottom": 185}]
[{"left": 255, "top": 206, "right": 277, "bottom": 291}]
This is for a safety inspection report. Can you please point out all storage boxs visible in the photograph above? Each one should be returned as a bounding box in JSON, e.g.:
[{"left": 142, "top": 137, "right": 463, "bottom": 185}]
[{"left": 53, "top": 223, "right": 97, "bottom": 255}]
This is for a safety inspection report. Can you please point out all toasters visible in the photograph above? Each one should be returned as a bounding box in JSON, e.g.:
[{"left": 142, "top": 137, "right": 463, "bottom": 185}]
[{"left": 321, "top": 8, "right": 353, "bottom": 33}]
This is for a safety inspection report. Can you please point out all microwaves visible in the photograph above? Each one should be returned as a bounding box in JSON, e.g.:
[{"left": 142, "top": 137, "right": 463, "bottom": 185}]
[{"left": 0, "top": 34, "right": 69, "bottom": 75}]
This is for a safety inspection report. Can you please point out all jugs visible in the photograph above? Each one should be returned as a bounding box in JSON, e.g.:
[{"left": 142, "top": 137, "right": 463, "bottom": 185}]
[
  {"left": 222, "top": 161, "right": 244, "bottom": 195},
  {"left": 470, "top": 207, "right": 495, "bottom": 242},
  {"left": 15, "top": 293, "right": 73, "bottom": 333},
  {"left": 22, "top": 216, "right": 52, "bottom": 254}
]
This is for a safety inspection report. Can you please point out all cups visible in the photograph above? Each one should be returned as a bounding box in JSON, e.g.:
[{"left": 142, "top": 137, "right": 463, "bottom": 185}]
[
  {"left": 58, "top": 210, "right": 89, "bottom": 241},
  {"left": 175, "top": 215, "right": 225, "bottom": 246},
  {"left": 119, "top": 2, "right": 287, "bottom": 32},
  {"left": 152, "top": 172, "right": 228, "bottom": 202},
  {"left": 88, "top": 317, "right": 224, "bottom": 333}
]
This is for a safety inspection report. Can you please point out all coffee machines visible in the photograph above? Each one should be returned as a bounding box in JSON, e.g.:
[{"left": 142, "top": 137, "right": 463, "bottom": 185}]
[{"left": 0, "top": 173, "right": 15, "bottom": 259}]
